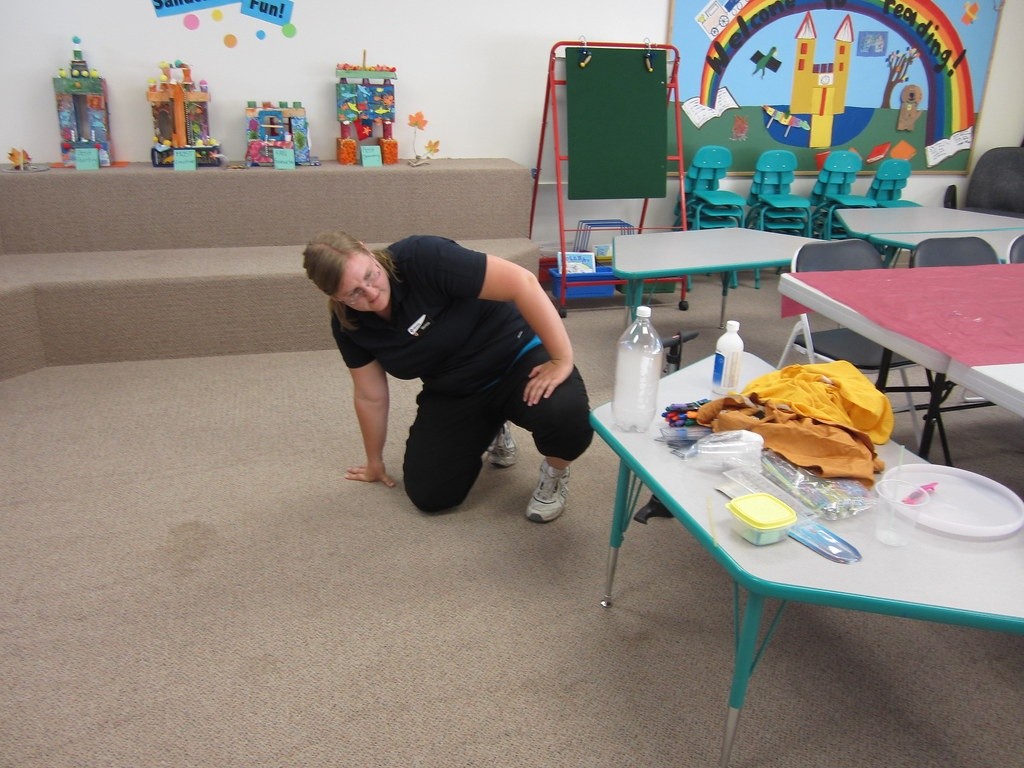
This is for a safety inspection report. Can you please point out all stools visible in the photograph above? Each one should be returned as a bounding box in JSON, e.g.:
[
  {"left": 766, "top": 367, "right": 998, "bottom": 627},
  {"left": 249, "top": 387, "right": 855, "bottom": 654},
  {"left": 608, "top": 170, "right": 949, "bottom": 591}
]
[{"left": 574, "top": 220, "right": 635, "bottom": 253}]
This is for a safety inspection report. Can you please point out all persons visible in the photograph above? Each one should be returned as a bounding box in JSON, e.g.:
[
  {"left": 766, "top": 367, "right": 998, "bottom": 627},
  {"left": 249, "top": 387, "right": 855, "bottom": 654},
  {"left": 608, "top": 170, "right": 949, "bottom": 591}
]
[{"left": 302, "top": 229, "right": 594, "bottom": 523}]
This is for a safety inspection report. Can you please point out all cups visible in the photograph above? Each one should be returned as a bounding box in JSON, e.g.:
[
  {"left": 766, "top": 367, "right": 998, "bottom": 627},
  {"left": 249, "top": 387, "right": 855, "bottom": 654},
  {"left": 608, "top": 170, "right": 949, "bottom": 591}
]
[{"left": 876, "top": 479, "right": 930, "bottom": 546}]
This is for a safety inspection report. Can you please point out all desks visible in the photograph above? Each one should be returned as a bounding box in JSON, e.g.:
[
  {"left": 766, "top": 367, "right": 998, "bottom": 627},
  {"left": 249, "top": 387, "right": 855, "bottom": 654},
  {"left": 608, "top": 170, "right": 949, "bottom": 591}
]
[
  {"left": 612, "top": 227, "right": 827, "bottom": 328},
  {"left": 778, "top": 262, "right": 1024, "bottom": 469},
  {"left": 866, "top": 228, "right": 1024, "bottom": 269},
  {"left": 588, "top": 351, "right": 1024, "bottom": 768},
  {"left": 833, "top": 207, "right": 1024, "bottom": 238}
]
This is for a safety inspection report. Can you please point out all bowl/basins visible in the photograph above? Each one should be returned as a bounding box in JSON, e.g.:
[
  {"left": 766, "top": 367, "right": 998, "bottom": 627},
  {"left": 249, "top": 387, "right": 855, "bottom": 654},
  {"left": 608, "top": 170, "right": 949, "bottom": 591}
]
[{"left": 726, "top": 492, "right": 797, "bottom": 545}]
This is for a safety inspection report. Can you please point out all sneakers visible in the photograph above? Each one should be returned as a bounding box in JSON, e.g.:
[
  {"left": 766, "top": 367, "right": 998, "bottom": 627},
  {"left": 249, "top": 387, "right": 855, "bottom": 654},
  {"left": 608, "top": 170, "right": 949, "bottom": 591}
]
[
  {"left": 485, "top": 422, "right": 519, "bottom": 467},
  {"left": 526, "top": 460, "right": 570, "bottom": 524}
]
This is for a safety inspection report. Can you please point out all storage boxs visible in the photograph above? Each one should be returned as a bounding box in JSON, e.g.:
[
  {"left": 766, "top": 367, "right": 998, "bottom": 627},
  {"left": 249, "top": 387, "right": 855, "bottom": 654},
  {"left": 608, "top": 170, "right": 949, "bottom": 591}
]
[{"left": 538, "top": 256, "right": 676, "bottom": 300}]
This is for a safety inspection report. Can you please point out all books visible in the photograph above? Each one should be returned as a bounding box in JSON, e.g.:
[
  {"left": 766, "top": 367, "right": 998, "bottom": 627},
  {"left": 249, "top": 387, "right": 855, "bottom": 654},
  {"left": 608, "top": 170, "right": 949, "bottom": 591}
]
[{"left": 558, "top": 252, "right": 596, "bottom": 274}]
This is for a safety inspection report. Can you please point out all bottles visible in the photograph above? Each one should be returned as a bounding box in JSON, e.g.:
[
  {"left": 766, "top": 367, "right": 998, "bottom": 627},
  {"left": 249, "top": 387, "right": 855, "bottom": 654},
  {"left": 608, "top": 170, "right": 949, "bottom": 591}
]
[
  {"left": 712, "top": 320, "right": 744, "bottom": 394},
  {"left": 611, "top": 306, "right": 663, "bottom": 432}
]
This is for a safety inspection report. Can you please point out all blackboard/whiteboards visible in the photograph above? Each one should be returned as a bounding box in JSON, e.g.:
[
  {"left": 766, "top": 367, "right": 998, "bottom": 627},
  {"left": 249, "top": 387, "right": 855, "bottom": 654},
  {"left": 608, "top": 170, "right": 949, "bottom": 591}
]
[{"left": 565, "top": 47, "right": 668, "bottom": 200}]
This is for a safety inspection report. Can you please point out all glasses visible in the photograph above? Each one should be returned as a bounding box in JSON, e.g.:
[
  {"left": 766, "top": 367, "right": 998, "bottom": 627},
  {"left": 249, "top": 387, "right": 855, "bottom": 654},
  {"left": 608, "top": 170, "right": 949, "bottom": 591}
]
[{"left": 342, "top": 261, "right": 383, "bottom": 308}]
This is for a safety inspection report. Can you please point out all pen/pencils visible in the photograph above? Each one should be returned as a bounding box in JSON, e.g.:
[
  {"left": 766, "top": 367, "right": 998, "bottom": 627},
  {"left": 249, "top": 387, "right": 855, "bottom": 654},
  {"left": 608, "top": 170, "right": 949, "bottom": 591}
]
[{"left": 661, "top": 399, "right": 712, "bottom": 428}]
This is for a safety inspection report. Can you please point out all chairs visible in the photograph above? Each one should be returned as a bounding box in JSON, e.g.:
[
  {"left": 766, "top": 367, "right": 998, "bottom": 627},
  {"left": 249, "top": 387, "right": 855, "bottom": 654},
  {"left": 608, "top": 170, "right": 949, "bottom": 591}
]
[{"left": 670, "top": 147, "right": 1024, "bottom": 469}]
